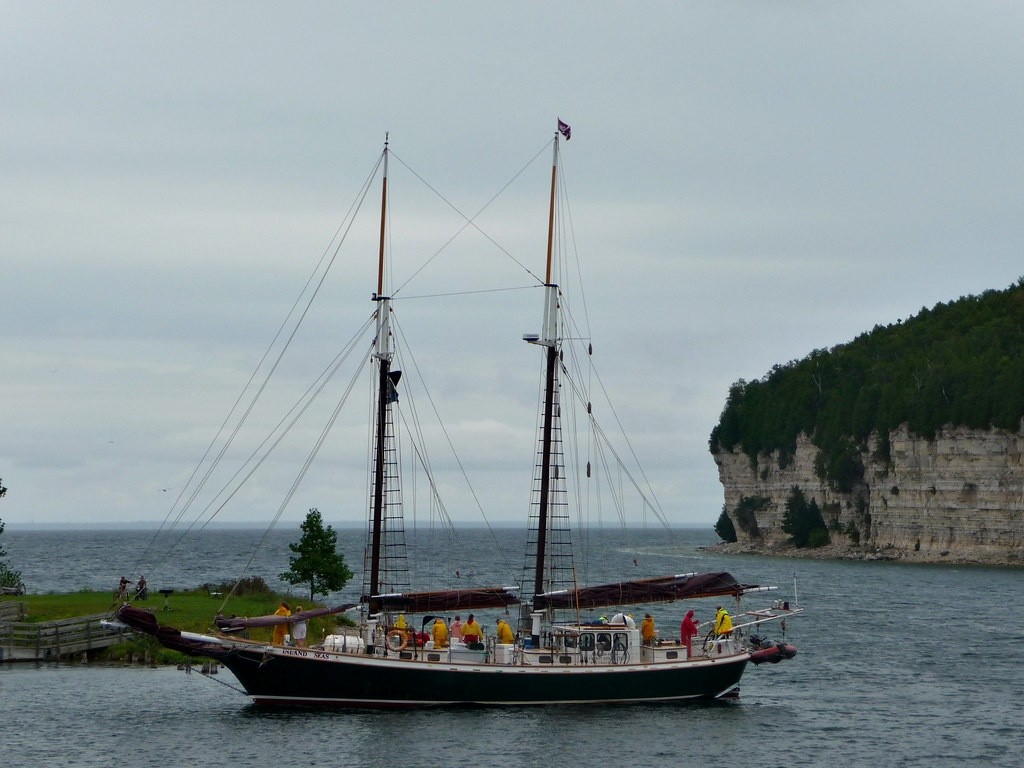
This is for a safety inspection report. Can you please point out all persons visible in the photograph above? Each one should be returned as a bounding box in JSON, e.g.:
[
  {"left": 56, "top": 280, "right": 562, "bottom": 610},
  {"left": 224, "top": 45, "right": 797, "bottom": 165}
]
[
  {"left": 138, "top": 576, "right": 146, "bottom": 598},
  {"left": 599, "top": 612, "right": 609, "bottom": 641},
  {"left": 641, "top": 614, "right": 654, "bottom": 643},
  {"left": 120, "top": 577, "right": 134, "bottom": 600},
  {"left": 432, "top": 619, "right": 448, "bottom": 646},
  {"left": 496, "top": 619, "right": 513, "bottom": 644},
  {"left": 713, "top": 606, "right": 732, "bottom": 640},
  {"left": 461, "top": 614, "right": 483, "bottom": 644},
  {"left": 293, "top": 606, "right": 309, "bottom": 647},
  {"left": 681, "top": 610, "right": 699, "bottom": 658},
  {"left": 450, "top": 616, "right": 463, "bottom": 643},
  {"left": 272, "top": 601, "right": 291, "bottom": 644}
]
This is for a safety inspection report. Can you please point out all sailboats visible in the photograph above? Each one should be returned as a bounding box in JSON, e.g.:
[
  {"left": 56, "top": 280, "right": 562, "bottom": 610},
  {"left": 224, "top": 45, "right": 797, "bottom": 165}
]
[{"left": 85, "top": 122, "right": 810, "bottom": 709}]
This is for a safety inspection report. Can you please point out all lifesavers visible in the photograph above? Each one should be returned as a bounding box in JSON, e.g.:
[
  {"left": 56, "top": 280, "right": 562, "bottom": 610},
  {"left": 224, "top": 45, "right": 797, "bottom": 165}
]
[{"left": 385, "top": 629, "right": 408, "bottom": 652}]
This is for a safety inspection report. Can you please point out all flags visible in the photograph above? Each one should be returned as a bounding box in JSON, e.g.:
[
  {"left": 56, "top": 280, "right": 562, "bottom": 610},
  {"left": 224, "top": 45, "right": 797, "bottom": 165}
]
[{"left": 559, "top": 121, "right": 571, "bottom": 140}]
